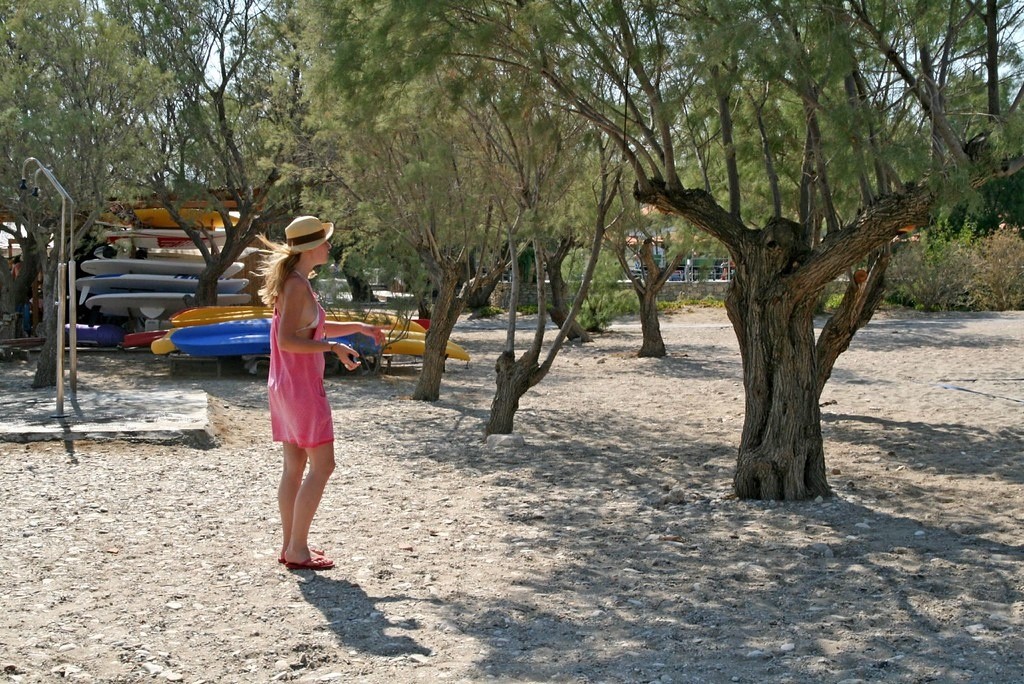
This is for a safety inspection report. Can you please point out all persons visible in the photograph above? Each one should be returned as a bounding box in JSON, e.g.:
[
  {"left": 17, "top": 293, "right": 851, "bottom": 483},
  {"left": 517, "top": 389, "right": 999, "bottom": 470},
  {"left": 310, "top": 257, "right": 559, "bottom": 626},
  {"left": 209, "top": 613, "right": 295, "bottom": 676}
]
[
  {"left": 634, "top": 254, "right": 640, "bottom": 269},
  {"left": 12, "top": 256, "right": 33, "bottom": 334},
  {"left": 256, "top": 215, "right": 392, "bottom": 570},
  {"left": 721, "top": 260, "right": 735, "bottom": 280}
]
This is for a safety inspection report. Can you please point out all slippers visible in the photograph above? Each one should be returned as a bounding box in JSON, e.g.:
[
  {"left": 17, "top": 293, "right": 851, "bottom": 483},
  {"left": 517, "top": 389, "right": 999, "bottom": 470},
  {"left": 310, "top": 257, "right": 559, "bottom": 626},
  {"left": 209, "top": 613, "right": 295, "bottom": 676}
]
[
  {"left": 278, "top": 549, "right": 324, "bottom": 564},
  {"left": 286, "top": 556, "right": 335, "bottom": 570}
]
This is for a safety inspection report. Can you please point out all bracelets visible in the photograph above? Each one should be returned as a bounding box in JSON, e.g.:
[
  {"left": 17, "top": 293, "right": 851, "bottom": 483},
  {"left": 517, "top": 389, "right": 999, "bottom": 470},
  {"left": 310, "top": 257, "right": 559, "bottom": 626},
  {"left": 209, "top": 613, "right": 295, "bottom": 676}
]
[{"left": 331, "top": 342, "right": 339, "bottom": 351}]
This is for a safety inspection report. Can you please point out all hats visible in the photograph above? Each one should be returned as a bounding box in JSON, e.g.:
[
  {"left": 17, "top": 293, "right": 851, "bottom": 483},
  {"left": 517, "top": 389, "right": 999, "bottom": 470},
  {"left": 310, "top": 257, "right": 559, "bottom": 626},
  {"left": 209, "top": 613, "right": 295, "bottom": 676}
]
[{"left": 282, "top": 216, "right": 335, "bottom": 252}]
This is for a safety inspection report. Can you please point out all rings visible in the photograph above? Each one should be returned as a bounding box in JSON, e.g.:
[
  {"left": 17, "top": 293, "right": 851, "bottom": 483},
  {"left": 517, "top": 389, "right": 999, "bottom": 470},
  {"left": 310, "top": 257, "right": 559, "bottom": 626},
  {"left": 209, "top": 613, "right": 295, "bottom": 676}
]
[{"left": 344, "top": 364, "right": 349, "bottom": 368}]
